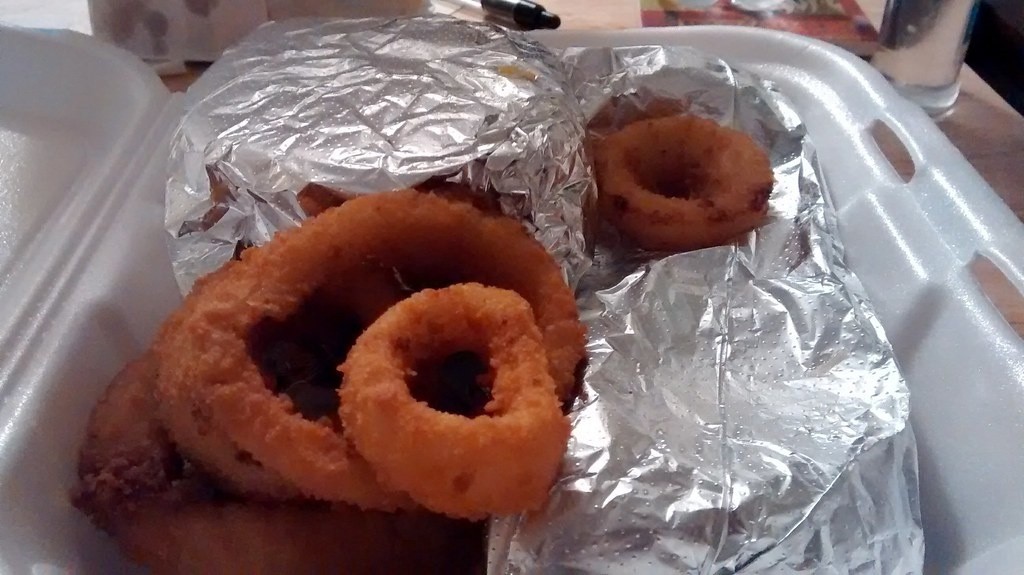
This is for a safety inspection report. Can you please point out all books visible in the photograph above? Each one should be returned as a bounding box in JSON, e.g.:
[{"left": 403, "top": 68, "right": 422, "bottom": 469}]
[{"left": 639, "top": 0, "right": 880, "bottom": 58}]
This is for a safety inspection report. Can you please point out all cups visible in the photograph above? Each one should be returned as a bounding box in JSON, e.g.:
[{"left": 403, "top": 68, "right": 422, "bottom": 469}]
[{"left": 871, "top": 1, "right": 975, "bottom": 126}]
